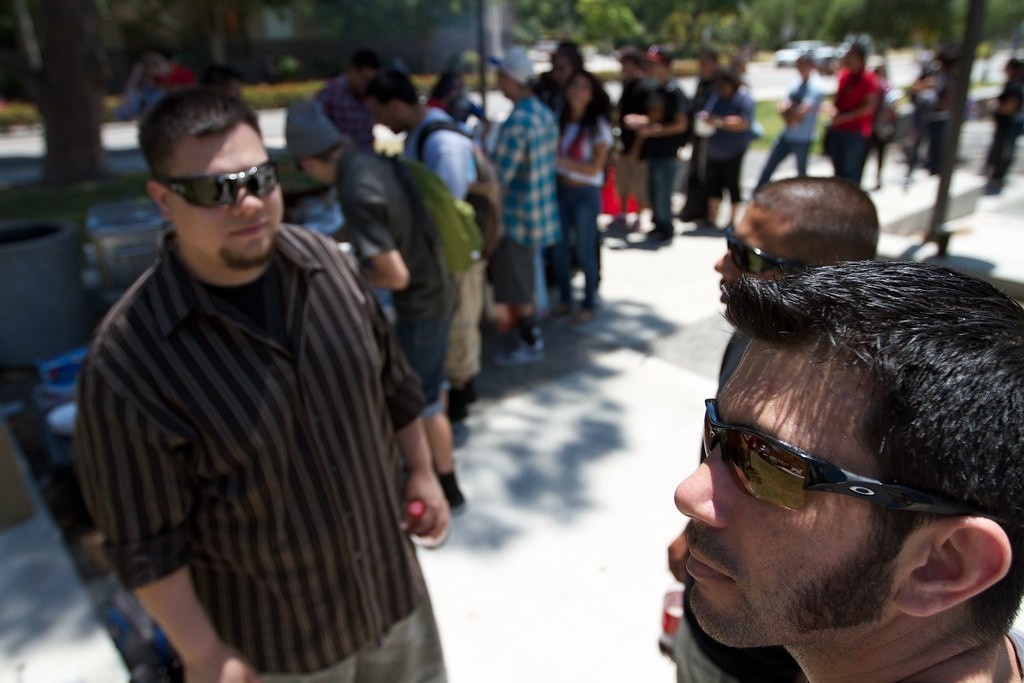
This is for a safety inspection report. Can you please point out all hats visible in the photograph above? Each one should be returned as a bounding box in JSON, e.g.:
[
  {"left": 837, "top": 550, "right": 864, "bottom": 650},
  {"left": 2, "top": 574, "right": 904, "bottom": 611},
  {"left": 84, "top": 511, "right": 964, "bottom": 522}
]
[
  {"left": 486, "top": 48, "right": 536, "bottom": 85},
  {"left": 283, "top": 98, "right": 339, "bottom": 156},
  {"left": 643, "top": 44, "right": 672, "bottom": 65}
]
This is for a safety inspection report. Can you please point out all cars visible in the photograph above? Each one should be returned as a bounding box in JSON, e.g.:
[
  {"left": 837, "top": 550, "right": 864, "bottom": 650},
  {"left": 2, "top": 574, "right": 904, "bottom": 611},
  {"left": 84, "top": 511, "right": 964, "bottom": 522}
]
[{"left": 773, "top": 39, "right": 828, "bottom": 66}]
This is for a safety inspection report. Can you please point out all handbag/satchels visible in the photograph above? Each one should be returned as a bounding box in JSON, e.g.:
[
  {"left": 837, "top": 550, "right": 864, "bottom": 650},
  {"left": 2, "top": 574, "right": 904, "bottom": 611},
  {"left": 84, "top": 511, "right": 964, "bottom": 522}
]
[{"left": 464, "top": 145, "right": 506, "bottom": 259}]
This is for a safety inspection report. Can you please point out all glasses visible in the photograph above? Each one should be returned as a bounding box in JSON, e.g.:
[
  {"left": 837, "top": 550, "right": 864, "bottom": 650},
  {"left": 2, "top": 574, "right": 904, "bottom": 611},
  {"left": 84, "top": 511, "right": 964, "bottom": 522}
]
[
  {"left": 723, "top": 222, "right": 818, "bottom": 276},
  {"left": 157, "top": 157, "right": 277, "bottom": 207},
  {"left": 698, "top": 397, "right": 974, "bottom": 517}
]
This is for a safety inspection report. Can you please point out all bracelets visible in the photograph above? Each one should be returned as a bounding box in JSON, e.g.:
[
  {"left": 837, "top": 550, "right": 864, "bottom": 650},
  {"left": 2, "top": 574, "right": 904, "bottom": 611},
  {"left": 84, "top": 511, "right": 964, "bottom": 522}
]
[{"left": 715, "top": 117, "right": 723, "bottom": 128}]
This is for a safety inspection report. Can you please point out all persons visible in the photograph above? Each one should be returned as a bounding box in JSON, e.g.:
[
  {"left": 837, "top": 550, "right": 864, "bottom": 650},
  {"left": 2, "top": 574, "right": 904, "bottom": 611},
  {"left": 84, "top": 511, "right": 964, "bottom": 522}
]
[
  {"left": 287, "top": 30, "right": 1024, "bottom": 509},
  {"left": 677, "top": 264, "right": 1024, "bottom": 682},
  {"left": 73, "top": 91, "right": 453, "bottom": 683},
  {"left": 669, "top": 176, "right": 880, "bottom": 683},
  {"left": 113, "top": 52, "right": 241, "bottom": 119}
]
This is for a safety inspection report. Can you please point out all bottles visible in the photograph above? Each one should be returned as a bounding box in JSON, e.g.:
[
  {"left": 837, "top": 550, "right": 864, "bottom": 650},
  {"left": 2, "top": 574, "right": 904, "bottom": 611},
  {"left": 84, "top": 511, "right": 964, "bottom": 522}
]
[
  {"left": 659, "top": 577, "right": 684, "bottom": 654},
  {"left": 406, "top": 499, "right": 448, "bottom": 549}
]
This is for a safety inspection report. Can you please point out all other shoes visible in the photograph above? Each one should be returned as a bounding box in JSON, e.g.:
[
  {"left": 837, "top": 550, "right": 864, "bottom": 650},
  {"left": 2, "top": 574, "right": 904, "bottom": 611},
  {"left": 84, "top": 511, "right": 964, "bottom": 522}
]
[
  {"left": 493, "top": 326, "right": 545, "bottom": 366},
  {"left": 444, "top": 489, "right": 464, "bottom": 508}
]
